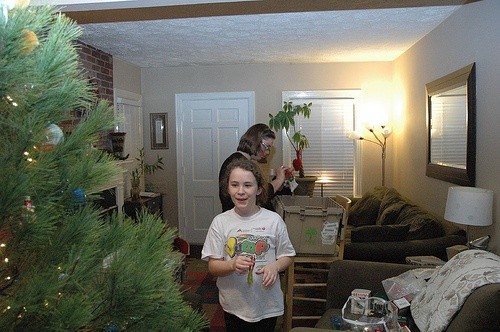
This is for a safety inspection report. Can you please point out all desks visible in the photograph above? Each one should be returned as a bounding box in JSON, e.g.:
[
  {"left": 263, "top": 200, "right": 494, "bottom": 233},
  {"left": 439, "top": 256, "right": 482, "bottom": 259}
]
[{"left": 123, "top": 191, "right": 164, "bottom": 225}]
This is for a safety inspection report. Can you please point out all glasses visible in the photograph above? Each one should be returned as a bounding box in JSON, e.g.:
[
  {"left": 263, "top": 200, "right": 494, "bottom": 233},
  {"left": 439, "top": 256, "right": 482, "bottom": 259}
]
[{"left": 262, "top": 142, "right": 272, "bottom": 151}]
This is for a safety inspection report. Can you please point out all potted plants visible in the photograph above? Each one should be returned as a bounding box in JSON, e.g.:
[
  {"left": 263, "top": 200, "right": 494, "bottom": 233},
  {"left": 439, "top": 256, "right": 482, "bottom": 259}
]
[
  {"left": 268, "top": 101, "right": 317, "bottom": 198},
  {"left": 128, "top": 146, "right": 166, "bottom": 201}
]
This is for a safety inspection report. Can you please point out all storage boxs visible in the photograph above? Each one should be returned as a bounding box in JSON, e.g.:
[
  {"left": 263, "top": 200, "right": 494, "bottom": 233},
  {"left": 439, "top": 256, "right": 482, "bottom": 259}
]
[
  {"left": 390, "top": 292, "right": 416, "bottom": 316},
  {"left": 274, "top": 195, "right": 346, "bottom": 256},
  {"left": 351, "top": 288, "right": 371, "bottom": 316}
]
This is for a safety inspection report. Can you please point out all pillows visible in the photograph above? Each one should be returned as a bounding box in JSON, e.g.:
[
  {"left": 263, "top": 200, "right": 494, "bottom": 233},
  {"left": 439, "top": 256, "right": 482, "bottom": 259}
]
[
  {"left": 376, "top": 188, "right": 407, "bottom": 226},
  {"left": 350, "top": 223, "right": 411, "bottom": 242},
  {"left": 347, "top": 192, "right": 381, "bottom": 226}
]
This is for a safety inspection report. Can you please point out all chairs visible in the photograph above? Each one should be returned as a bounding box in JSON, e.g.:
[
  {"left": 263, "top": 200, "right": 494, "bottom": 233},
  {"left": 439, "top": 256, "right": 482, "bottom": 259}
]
[{"left": 285, "top": 194, "right": 351, "bottom": 331}]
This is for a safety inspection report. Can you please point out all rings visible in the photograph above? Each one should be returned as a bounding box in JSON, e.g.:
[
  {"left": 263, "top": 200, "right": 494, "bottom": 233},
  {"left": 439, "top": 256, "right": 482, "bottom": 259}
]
[{"left": 281, "top": 165, "right": 284, "bottom": 168}]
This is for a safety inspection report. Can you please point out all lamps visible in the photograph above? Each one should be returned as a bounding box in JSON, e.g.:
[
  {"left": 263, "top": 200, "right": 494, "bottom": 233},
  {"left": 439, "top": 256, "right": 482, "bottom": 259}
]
[
  {"left": 347, "top": 116, "right": 393, "bottom": 187},
  {"left": 443, "top": 185, "right": 494, "bottom": 251}
]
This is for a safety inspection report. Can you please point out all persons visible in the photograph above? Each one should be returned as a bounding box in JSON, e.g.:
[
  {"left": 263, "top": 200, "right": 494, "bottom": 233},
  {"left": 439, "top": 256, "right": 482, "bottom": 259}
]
[
  {"left": 218, "top": 123, "right": 294, "bottom": 214},
  {"left": 200, "top": 159, "right": 296, "bottom": 332}
]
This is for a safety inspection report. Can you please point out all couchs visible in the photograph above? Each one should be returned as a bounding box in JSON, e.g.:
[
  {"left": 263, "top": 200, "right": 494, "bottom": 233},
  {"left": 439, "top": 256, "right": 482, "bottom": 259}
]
[
  {"left": 343, "top": 195, "right": 467, "bottom": 266},
  {"left": 288, "top": 260, "right": 500, "bottom": 331}
]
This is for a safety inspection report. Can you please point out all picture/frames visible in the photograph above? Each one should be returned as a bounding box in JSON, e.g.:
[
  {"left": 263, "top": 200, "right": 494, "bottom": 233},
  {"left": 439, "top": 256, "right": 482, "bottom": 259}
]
[{"left": 150, "top": 113, "right": 169, "bottom": 150}]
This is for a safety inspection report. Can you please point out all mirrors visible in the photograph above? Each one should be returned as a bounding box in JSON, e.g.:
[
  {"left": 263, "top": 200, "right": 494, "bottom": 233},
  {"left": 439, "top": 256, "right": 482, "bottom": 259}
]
[{"left": 424, "top": 62, "right": 476, "bottom": 188}]
